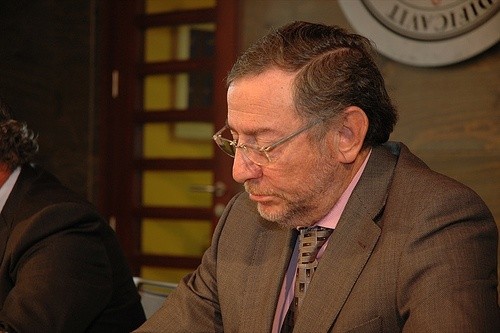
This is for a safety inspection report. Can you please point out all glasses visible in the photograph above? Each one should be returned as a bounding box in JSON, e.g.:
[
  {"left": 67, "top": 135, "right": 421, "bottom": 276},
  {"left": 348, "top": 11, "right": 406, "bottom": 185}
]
[{"left": 212, "top": 108, "right": 346, "bottom": 166}]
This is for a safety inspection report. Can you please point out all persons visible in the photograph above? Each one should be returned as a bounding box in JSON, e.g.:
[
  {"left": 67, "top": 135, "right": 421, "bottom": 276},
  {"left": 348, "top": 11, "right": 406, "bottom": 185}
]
[
  {"left": 129, "top": 20, "right": 500, "bottom": 333},
  {"left": 0, "top": 99, "right": 147, "bottom": 333}
]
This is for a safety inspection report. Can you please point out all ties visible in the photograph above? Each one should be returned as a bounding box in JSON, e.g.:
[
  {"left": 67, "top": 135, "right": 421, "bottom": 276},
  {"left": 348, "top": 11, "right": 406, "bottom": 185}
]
[{"left": 281, "top": 226, "right": 334, "bottom": 333}]
